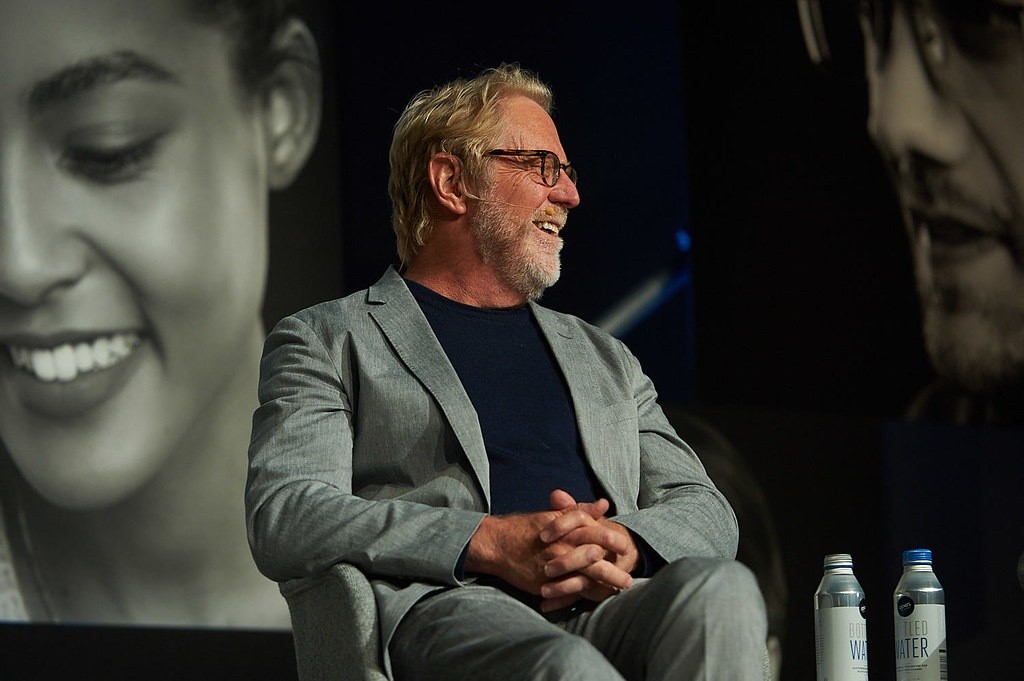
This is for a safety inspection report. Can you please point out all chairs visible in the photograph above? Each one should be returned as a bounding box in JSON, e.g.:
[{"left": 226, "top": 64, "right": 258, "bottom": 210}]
[{"left": 278, "top": 562, "right": 391, "bottom": 681}]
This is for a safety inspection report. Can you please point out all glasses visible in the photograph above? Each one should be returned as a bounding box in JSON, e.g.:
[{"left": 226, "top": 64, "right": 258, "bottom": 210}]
[{"left": 452, "top": 149, "right": 577, "bottom": 186}]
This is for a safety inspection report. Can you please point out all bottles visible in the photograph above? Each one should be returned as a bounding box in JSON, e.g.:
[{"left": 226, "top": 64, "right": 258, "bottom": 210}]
[
  {"left": 894, "top": 549, "right": 948, "bottom": 681},
  {"left": 814, "top": 553, "right": 869, "bottom": 681}
]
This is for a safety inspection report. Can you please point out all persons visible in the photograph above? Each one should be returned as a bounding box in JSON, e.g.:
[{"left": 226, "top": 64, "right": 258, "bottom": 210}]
[
  {"left": 856, "top": 0, "right": 1024, "bottom": 381},
  {"left": 0, "top": 0, "right": 326, "bottom": 627},
  {"left": 245, "top": 72, "right": 770, "bottom": 680}
]
